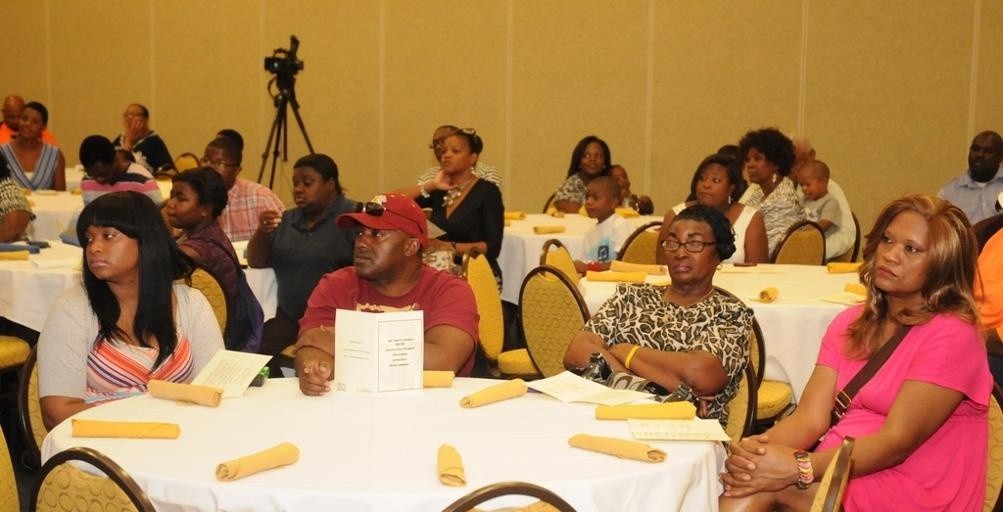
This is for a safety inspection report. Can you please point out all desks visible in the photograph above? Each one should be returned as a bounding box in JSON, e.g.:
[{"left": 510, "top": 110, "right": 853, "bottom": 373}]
[
  {"left": 42, "top": 376, "right": 730, "bottom": 511},
  {"left": 232, "top": 237, "right": 277, "bottom": 324},
  {"left": 1, "top": 243, "right": 86, "bottom": 332},
  {"left": 24, "top": 189, "right": 85, "bottom": 242},
  {"left": 61, "top": 163, "right": 85, "bottom": 192}
]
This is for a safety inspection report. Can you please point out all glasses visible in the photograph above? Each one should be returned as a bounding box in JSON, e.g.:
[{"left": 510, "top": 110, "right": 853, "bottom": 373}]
[
  {"left": 659, "top": 237, "right": 717, "bottom": 253},
  {"left": 352, "top": 201, "right": 424, "bottom": 236},
  {"left": 449, "top": 127, "right": 479, "bottom": 150},
  {"left": 199, "top": 156, "right": 237, "bottom": 171}
]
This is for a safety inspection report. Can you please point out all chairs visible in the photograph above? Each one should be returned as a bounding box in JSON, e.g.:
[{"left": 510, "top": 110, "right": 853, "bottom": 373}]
[
  {"left": 18, "top": 343, "right": 49, "bottom": 459},
  {"left": 1, "top": 427, "right": 25, "bottom": 511},
  {"left": 0, "top": 333, "right": 32, "bottom": 378},
  {"left": 178, "top": 270, "right": 229, "bottom": 336},
  {"left": 29, "top": 447, "right": 158, "bottom": 511},
  {"left": 174, "top": 151, "right": 199, "bottom": 176}
]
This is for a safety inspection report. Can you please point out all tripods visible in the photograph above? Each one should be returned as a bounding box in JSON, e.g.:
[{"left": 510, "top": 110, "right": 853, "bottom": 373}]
[{"left": 253, "top": 89, "right": 340, "bottom": 195}]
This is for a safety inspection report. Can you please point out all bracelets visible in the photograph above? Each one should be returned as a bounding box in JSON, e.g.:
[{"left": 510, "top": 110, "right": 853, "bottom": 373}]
[
  {"left": 625, "top": 344, "right": 640, "bottom": 371},
  {"left": 793, "top": 448, "right": 814, "bottom": 490},
  {"left": 418, "top": 184, "right": 431, "bottom": 199}
]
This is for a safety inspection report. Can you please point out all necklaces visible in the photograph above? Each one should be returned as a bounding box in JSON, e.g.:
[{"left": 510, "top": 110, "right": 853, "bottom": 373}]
[{"left": 441, "top": 175, "right": 474, "bottom": 209}]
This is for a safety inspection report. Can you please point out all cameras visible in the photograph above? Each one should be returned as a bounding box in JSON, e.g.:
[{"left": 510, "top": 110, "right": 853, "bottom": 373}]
[{"left": 260, "top": 34, "right": 308, "bottom": 88}]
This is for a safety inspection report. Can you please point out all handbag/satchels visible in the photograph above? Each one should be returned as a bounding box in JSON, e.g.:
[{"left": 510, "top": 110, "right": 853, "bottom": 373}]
[
  {"left": 567, "top": 352, "right": 700, "bottom": 410},
  {"left": 189, "top": 234, "right": 266, "bottom": 352}
]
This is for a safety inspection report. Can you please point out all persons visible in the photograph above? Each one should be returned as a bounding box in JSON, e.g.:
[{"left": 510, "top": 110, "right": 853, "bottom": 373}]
[
  {"left": 246, "top": 152, "right": 369, "bottom": 378},
  {"left": 716, "top": 192, "right": 995, "bottom": 512},
  {"left": 552, "top": 135, "right": 654, "bottom": 264},
  {"left": 657, "top": 125, "right": 858, "bottom": 265},
  {"left": 290, "top": 191, "right": 481, "bottom": 398},
  {"left": 416, "top": 124, "right": 504, "bottom": 197},
  {"left": 382, "top": 129, "right": 505, "bottom": 295},
  {"left": 938, "top": 130, "right": 1003, "bottom": 228},
  {"left": 562, "top": 203, "right": 754, "bottom": 446},
  {"left": 163, "top": 165, "right": 263, "bottom": 352},
  {"left": 36, "top": 189, "right": 227, "bottom": 434},
  {"left": 0, "top": 95, "right": 176, "bottom": 246},
  {"left": 157, "top": 127, "right": 286, "bottom": 241}
]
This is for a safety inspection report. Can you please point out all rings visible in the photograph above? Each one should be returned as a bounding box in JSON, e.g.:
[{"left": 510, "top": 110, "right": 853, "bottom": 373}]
[{"left": 263, "top": 220, "right": 268, "bottom": 226}]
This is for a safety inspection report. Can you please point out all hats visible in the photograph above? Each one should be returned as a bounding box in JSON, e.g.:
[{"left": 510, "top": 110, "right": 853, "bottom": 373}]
[{"left": 336, "top": 191, "right": 429, "bottom": 251}]
